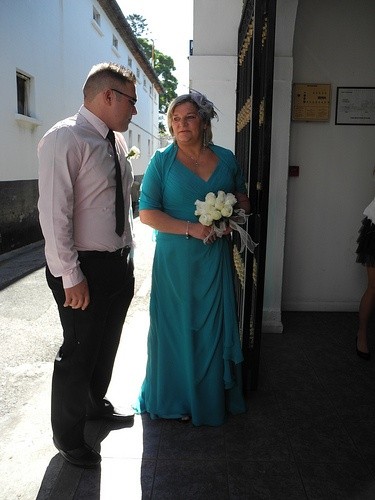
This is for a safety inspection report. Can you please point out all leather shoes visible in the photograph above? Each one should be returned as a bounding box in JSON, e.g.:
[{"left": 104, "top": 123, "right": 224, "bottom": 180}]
[
  {"left": 86, "top": 405, "right": 135, "bottom": 423},
  {"left": 53, "top": 437, "right": 102, "bottom": 465}
]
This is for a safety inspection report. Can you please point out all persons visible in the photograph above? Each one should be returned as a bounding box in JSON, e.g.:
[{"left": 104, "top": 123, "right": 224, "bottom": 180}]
[
  {"left": 354, "top": 198, "right": 375, "bottom": 360},
  {"left": 137, "top": 87, "right": 252, "bottom": 427},
  {"left": 36, "top": 62, "right": 137, "bottom": 468}
]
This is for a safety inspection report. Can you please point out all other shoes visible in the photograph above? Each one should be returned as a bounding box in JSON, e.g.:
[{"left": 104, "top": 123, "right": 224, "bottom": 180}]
[{"left": 356, "top": 334, "right": 370, "bottom": 359}]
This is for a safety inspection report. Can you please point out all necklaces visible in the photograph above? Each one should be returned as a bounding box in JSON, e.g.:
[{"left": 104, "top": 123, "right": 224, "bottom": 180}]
[{"left": 179, "top": 147, "right": 205, "bottom": 167}]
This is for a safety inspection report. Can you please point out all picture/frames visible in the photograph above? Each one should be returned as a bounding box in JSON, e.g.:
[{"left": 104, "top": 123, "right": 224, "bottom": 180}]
[{"left": 335, "top": 87, "right": 375, "bottom": 125}]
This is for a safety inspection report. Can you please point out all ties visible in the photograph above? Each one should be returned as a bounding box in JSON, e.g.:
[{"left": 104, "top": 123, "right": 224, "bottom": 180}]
[{"left": 107, "top": 128, "right": 125, "bottom": 237}]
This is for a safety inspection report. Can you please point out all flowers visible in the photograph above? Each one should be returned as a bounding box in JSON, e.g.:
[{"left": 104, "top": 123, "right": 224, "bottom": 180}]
[
  {"left": 195, "top": 191, "right": 259, "bottom": 254},
  {"left": 126, "top": 146, "right": 140, "bottom": 159}
]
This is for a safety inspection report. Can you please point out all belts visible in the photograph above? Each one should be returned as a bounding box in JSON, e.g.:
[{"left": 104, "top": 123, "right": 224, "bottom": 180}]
[{"left": 77, "top": 247, "right": 130, "bottom": 257}]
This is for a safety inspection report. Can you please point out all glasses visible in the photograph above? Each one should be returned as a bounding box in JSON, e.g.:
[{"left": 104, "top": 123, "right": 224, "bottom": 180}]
[{"left": 110, "top": 89, "right": 137, "bottom": 105}]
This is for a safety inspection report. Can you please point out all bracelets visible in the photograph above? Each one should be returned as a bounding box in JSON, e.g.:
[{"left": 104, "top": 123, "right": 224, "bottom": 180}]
[{"left": 186, "top": 221, "right": 191, "bottom": 241}]
